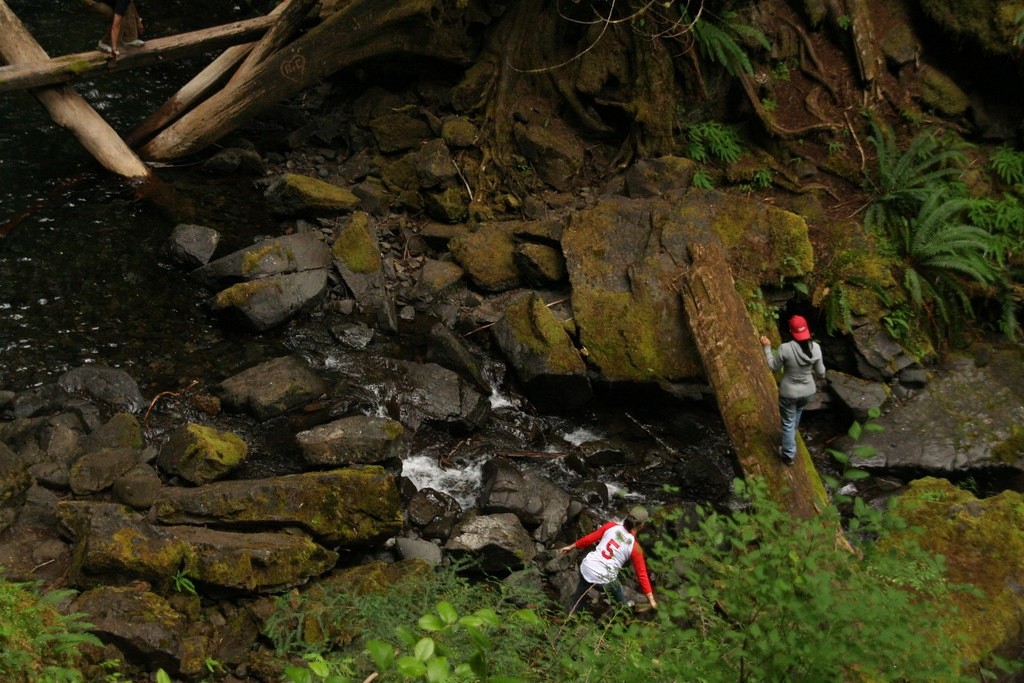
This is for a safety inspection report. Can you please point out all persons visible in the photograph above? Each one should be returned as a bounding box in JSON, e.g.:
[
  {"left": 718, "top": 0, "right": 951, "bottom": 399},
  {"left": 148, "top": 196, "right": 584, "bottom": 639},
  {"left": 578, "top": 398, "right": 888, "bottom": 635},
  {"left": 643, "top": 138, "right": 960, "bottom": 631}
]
[
  {"left": 761, "top": 316, "right": 826, "bottom": 465},
  {"left": 560, "top": 505, "right": 656, "bottom": 621},
  {"left": 80, "top": 0, "right": 145, "bottom": 58}
]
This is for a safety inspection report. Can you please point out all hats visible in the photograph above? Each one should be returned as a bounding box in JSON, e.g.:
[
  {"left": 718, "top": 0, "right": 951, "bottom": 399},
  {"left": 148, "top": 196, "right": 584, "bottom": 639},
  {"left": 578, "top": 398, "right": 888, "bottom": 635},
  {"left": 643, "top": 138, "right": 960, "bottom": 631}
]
[
  {"left": 626, "top": 505, "right": 648, "bottom": 523},
  {"left": 789, "top": 316, "right": 811, "bottom": 340}
]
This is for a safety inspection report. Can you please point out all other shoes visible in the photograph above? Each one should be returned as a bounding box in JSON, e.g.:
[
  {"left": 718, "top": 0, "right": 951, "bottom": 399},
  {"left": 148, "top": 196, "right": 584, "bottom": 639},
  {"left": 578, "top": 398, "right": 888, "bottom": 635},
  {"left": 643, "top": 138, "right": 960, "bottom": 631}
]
[
  {"left": 628, "top": 600, "right": 635, "bottom": 607},
  {"left": 778, "top": 446, "right": 794, "bottom": 465}
]
